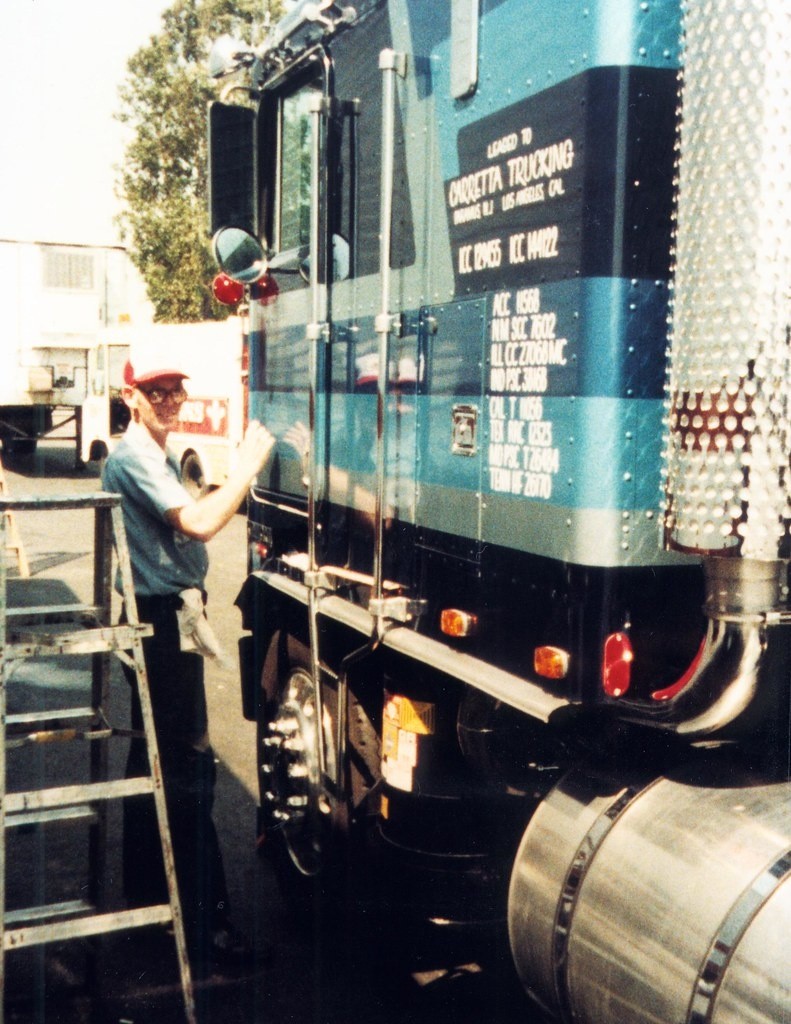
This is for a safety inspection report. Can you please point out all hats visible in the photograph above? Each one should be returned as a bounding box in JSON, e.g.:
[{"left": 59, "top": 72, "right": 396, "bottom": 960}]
[{"left": 122, "top": 357, "right": 191, "bottom": 386}]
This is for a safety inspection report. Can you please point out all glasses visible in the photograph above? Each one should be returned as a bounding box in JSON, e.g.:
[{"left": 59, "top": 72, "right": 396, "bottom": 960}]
[{"left": 136, "top": 383, "right": 188, "bottom": 405}]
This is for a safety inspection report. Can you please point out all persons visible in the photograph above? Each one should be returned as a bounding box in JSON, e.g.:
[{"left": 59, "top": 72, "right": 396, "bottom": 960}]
[
  {"left": 283, "top": 419, "right": 394, "bottom": 521},
  {"left": 102, "top": 346, "right": 275, "bottom": 960}
]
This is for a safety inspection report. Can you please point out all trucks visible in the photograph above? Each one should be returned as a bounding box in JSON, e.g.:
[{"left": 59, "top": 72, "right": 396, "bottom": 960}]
[
  {"left": 1, "top": 237, "right": 158, "bottom": 472},
  {"left": 203, "top": 0, "right": 790, "bottom": 1024}
]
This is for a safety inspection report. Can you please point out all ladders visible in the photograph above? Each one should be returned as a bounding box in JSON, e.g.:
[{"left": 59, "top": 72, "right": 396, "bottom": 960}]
[{"left": 0, "top": 490, "right": 199, "bottom": 1024}]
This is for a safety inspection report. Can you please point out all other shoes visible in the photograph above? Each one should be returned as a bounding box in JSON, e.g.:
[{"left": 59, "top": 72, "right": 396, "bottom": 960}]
[{"left": 183, "top": 917, "right": 252, "bottom": 961}]
